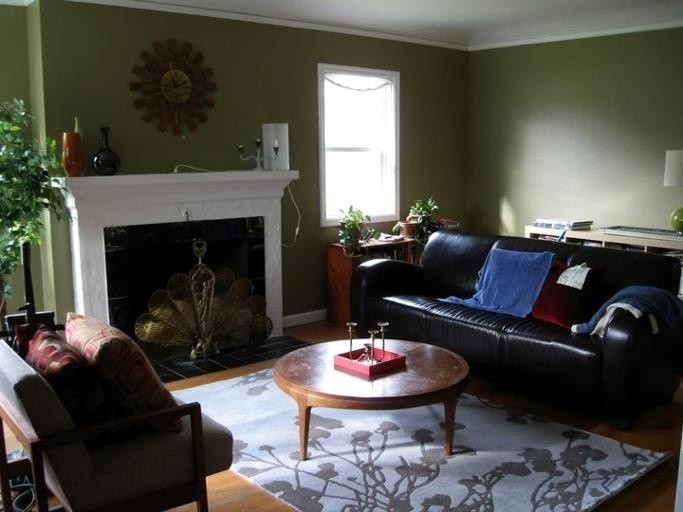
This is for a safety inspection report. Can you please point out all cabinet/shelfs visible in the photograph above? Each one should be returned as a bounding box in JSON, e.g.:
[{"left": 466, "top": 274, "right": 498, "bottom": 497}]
[
  {"left": 523, "top": 223, "right": 682, "bottom": 298},
  {"left": 326, "top": 233, "right": 413, "bottom": 328}
]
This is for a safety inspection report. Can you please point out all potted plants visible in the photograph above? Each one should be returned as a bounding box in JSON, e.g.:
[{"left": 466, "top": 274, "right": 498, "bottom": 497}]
[
  {"left": 392, "top": 192, "right": 440, "bottom": 237},
  {"left": 336, "top": 205, "right": 376, "bottom": 257}
]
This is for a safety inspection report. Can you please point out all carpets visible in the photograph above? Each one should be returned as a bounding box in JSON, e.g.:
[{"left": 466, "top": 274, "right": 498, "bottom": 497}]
[{"left": 170, "top": 344, "right": 672, "bottom": 511}]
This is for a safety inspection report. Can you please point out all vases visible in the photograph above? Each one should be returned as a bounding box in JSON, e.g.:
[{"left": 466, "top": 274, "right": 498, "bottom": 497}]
[
  {"left": 72, "top": 112, "right": 96, "bottom": 176},
  {"left": 90, "top": 126, "right": 119, "bottom": 176},
  {"left": 59, "top": 132, "right": 83, "bottom": 178}
]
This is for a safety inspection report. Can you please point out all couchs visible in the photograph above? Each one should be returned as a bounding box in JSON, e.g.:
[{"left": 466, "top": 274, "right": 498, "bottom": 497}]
[{"left": 350, "top": 229, "right": 682, "bottom": 426}]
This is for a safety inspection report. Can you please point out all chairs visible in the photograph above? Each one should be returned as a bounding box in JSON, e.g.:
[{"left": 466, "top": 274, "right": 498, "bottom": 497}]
[{"left": 0, "top": 322, "right": 233, "bottom": 512}]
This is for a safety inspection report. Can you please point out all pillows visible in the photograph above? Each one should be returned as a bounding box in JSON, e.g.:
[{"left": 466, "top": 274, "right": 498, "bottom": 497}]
[
  {"left": 24, "top": 323, "right": 137, "bottom": 448},
  {"left": 532, "top": 265, "right": 609, "bottom": 331},
  {"left": 64, "top": 311, "right": 183, "bottom": 435}
]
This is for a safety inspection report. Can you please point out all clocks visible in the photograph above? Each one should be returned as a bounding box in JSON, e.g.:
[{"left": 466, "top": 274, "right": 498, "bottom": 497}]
[{"left": 128, "top": 37, "right": 218, "bottom": 135}]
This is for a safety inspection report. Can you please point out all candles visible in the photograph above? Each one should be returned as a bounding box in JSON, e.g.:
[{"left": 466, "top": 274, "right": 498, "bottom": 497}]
[{"left": 273, "top": 139, "right": 278, "bottom": 147}]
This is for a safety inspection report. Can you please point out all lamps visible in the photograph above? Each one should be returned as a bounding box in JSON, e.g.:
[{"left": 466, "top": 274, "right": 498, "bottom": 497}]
[{"left": 661, "top": 149, "right": 682, "bottom": 234}]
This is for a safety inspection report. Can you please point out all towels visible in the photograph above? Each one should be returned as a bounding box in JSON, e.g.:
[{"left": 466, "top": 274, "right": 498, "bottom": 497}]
[{"left": 436, "top": 246, "right": 556, "bottom": 319}]
